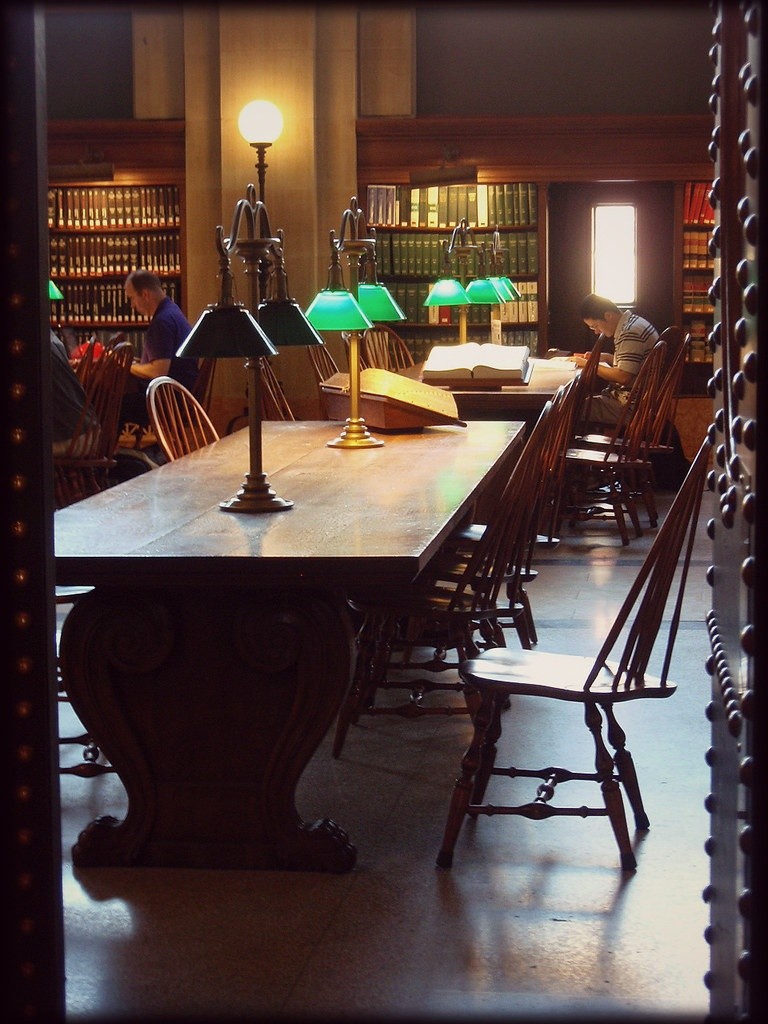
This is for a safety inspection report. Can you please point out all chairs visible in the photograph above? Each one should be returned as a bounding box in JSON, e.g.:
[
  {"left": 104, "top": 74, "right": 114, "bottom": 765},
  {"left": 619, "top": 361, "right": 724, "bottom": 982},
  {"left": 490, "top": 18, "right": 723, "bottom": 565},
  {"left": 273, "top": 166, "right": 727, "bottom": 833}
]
[{"left": 53, "top": 323, "right": 710, "bottom": 873}]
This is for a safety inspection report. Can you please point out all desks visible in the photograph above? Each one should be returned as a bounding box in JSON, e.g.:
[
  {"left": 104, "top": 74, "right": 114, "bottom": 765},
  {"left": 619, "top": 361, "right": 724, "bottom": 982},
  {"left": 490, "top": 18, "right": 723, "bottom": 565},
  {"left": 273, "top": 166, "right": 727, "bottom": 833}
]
[
  {"left": 392, "top": 358, "right": 584, "bottom": 524},
  {"left": 54, "top": 420, "right": 526, "bottom": 876}
]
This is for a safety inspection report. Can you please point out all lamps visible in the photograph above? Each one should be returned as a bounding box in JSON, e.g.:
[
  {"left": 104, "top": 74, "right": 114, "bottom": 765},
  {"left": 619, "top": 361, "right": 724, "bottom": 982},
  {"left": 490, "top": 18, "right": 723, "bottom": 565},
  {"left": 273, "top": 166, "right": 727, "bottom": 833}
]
[
  {"left": 176, "top": 182, "right": 325, "bottom": 513},
  {"left": 225, "top": 99, "right": 292, "bottom": 437},
  {"left": 304, "top": 196, "right": 407, "bottom": 449},
  {"left": 479, "top": 224, "right": 522, "bottom": 344},
  {"left": 423, "top": 217, "right": 503, "bottom": 345}
]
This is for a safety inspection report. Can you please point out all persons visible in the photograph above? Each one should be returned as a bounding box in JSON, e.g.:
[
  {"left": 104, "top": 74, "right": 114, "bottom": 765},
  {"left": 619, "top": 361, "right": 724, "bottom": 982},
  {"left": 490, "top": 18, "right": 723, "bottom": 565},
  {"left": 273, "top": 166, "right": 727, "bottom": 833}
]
[
  {"left": 564, "top": 292, "right": 663, "bottom": 424},
  {"left": 51, "top": 328, "right": 92, "bottom": 440},
  {"left": 104, "top": 268, "right": 204, "bottom": 430}
]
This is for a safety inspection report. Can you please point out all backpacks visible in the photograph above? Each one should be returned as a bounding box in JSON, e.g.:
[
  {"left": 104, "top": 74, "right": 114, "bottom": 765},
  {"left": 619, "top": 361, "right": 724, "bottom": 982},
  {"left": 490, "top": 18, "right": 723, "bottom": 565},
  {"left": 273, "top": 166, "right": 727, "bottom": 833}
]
[{"left": 641, "top": 418, "right": 691, "bottom": 491}]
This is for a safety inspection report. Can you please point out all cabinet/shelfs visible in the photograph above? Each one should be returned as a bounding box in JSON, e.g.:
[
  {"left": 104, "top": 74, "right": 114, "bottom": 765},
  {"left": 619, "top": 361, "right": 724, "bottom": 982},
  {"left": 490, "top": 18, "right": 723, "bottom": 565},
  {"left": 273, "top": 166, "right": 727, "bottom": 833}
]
[
  {"left": 45, "top": 118, "right": 188, "bottom": 333},
  {"left": 355, "top": 112, "right": 716, "bottom": 358}
]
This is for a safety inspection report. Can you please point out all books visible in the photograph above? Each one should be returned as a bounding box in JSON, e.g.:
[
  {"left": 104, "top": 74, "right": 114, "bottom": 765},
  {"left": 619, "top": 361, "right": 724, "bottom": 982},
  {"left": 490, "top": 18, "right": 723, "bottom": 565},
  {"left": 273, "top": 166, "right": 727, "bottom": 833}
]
[
  {"left": 45, "top": 187, "right": 179, "bottom": 228},
  {"left": 367, "top": 232, "right": 539, "bottom": 275},
  {"left": 387, "top": 329, "right": 539, "bottom": 369},
  {"left": 533, "top": 356, "right": 581, "bottom": 372},
  {"left": 55, "top": 330, "right": 147, "bottom": 362},
  {"left": 50, "top": 283, "right": 176, "bottom": 322},
  {"left": 422, "top": 341, "right": 530, "bottom": 379},
  {"left": 376, "top": 281, "right": 538, "bottom": 324},
  {"left": 365, "top": 183, "right": 539, "bottom": 228},
  {"left": 682, "top": 182, "right": 717, "bottom": 362},
  {"left": 49, "top": 235, "right": 179, "bottom": 274}
]
